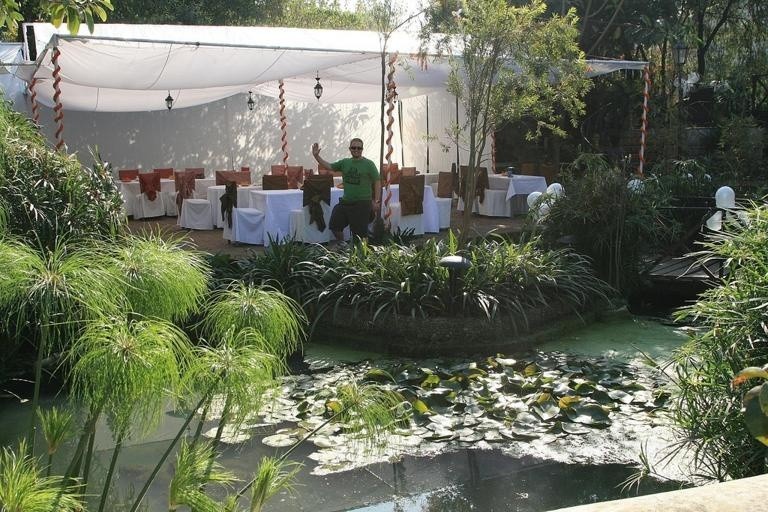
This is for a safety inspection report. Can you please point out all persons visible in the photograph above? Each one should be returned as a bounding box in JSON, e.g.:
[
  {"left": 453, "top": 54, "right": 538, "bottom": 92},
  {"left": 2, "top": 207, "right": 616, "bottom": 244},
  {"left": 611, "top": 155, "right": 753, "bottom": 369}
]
[{"left": 312, "top": 138, "right": 381, "bottom": 249}]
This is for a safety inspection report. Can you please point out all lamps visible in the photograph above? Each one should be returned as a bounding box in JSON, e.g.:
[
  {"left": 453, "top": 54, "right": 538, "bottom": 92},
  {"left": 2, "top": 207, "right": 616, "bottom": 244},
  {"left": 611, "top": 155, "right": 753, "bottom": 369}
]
[
  {"left": 246, "top": 91, "right": 255, "bottom": 111},
  {"left": 314, "top": 70, "right": 323, "bottom": 102},
  {"left": 166, "top": 90, "right": 174, "bottom": 110}
]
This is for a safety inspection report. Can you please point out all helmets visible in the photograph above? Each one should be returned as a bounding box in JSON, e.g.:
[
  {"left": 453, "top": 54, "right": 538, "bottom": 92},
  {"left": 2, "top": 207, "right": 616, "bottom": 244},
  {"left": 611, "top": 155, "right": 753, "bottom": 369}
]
[{"left": 350, "top": 146, "right": 364, "bottom": 151}]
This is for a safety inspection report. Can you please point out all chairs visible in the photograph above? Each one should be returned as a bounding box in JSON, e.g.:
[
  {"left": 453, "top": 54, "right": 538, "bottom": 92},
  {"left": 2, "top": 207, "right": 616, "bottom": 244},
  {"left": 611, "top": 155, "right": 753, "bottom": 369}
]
[{"left": 113, "top": 163, "right": 509, "bottom": 245}]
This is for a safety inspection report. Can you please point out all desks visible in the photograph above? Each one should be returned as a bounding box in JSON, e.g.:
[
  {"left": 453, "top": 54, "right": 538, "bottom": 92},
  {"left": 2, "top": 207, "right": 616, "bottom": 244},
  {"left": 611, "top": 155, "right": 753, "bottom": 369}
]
[{"left": 486, "top": 173, "right": 549, "bottom": 215}]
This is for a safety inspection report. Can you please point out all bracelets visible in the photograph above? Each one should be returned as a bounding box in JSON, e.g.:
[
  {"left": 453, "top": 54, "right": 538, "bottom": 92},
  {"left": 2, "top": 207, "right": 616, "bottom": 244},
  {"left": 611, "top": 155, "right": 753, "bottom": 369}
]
[{"left": 375, "top": 200, "right": 381, "bottom": 203}]
